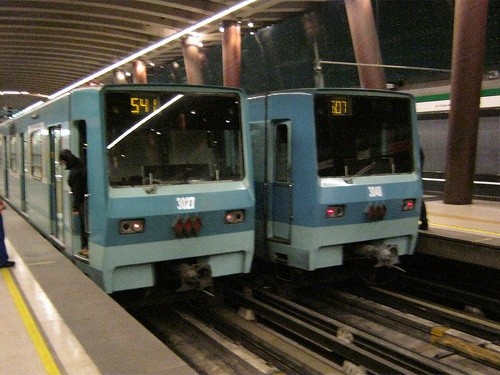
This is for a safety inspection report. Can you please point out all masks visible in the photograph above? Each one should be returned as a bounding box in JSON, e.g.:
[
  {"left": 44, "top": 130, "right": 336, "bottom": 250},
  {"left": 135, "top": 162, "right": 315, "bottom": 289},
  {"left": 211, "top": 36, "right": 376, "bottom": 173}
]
[{"left": 62, "top": 163, "right": 67, "bottom": 170}]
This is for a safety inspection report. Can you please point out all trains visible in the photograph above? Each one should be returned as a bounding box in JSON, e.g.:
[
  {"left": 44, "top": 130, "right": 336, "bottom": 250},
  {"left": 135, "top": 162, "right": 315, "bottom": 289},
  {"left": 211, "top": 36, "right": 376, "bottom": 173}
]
[
  {"left": 0, "top": 82, "right": 256, "bottom": 308},
  {"left": 241, "top": 87, "right": 420, "bottom": 298}
]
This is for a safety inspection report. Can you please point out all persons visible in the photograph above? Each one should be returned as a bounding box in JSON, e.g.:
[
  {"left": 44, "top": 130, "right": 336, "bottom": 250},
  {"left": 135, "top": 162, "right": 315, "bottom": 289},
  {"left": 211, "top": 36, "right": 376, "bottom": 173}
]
[
  {"left": 0, "top": 199, "right": 16, "bottom": 269},
  {"left": 59, "top": 147, "right": 88, "bottom": 256}
]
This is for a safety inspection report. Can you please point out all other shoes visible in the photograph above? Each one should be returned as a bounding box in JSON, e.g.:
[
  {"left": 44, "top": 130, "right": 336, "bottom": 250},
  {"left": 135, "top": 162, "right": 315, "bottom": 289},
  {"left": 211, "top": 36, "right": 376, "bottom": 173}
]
[
  {"left": 79, "top": 247, "right": 87, "bottom": 254},
  {"left": 82, "top": 250, "right": 88, "bottom": 255}
]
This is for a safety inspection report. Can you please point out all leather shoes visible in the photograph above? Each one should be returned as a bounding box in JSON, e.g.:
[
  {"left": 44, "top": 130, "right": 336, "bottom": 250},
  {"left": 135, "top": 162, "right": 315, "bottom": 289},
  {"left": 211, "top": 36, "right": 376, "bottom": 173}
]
[
  {"left": 0, "top": 261, "right": 15, "bottom": 269},
  {"left": 418, "top": 221, "right": 428, "bottom": 230}
]
